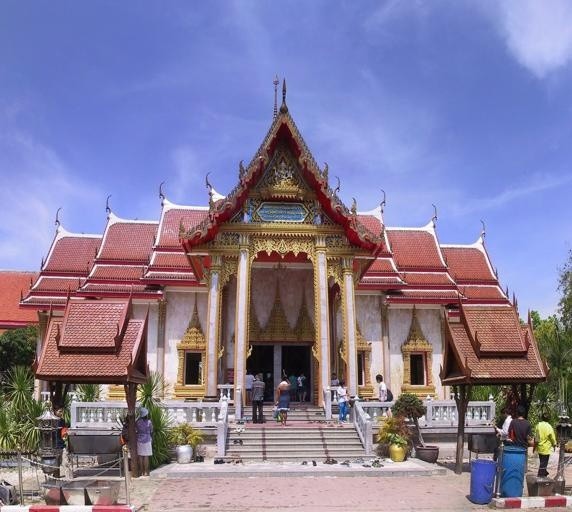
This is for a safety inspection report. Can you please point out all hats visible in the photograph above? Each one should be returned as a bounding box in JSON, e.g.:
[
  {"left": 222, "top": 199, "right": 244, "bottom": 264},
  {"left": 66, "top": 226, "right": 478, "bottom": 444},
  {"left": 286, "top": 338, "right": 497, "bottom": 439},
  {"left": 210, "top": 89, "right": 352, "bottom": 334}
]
[{"left": 140, "top": 407, "right": 149, "bottom": 418}]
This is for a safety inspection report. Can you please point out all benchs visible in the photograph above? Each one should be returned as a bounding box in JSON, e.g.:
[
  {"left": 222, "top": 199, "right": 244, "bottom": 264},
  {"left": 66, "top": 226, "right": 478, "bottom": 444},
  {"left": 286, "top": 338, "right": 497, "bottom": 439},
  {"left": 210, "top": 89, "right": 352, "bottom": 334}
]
[
  {"left": 468, "top": 432, "right": 499, "bottom": 472},
  {"left": 68, "top": 435, "right": 126, "bottom": 478}
]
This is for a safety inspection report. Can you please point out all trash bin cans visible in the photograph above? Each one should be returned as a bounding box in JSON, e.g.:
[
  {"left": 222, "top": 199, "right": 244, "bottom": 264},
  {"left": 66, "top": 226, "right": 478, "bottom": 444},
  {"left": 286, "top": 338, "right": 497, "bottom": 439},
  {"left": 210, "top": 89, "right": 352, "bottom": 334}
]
[
  {"left": 470, "top": 459, "right": 496, "bottom": 504},
  {"left": 500, "top": 446, "right": 527, "bottom": 497}
]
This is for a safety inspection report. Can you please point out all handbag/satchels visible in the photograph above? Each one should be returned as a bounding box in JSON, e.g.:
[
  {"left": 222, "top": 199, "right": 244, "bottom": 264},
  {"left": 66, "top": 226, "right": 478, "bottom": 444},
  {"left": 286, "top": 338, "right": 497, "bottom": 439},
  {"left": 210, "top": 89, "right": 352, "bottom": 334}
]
[{"left": 387, "top": 390, "right": 393, "bottom": 402}]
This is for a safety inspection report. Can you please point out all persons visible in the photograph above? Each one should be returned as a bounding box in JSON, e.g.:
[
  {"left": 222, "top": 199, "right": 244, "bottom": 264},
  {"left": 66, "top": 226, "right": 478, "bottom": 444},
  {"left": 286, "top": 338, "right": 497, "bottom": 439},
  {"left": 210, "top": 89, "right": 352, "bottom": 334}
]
[
  {"left": 135, "top": 407, "right": 153, "bottom": 476},
  {"left": 120, "top": 412, "right": 137, "bottom": 470},
  {"left": 48, "top": 404, "right": 66, "bottom": 478},
  {"left": 245, "top": 369, "right": 351, "bottom": 426},
  {"left": 376, "top": 374, "right": 391, "bottom": 417},
  {"left": 494, "top": 408, "right": 556, "bottom": 477}
]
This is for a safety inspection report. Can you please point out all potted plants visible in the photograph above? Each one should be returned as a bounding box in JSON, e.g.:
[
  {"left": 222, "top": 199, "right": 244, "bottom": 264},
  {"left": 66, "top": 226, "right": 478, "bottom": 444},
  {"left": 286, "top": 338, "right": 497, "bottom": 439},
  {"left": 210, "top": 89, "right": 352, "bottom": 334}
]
[
  {"left": 390, "top": 392, "right": 438, "bottom": 463},
  {"left": 375, "top": 410, "right": 416, "bottom": 462},
  {"left": 168, "top": 421, "right": 208, "bottom": 463}
]
